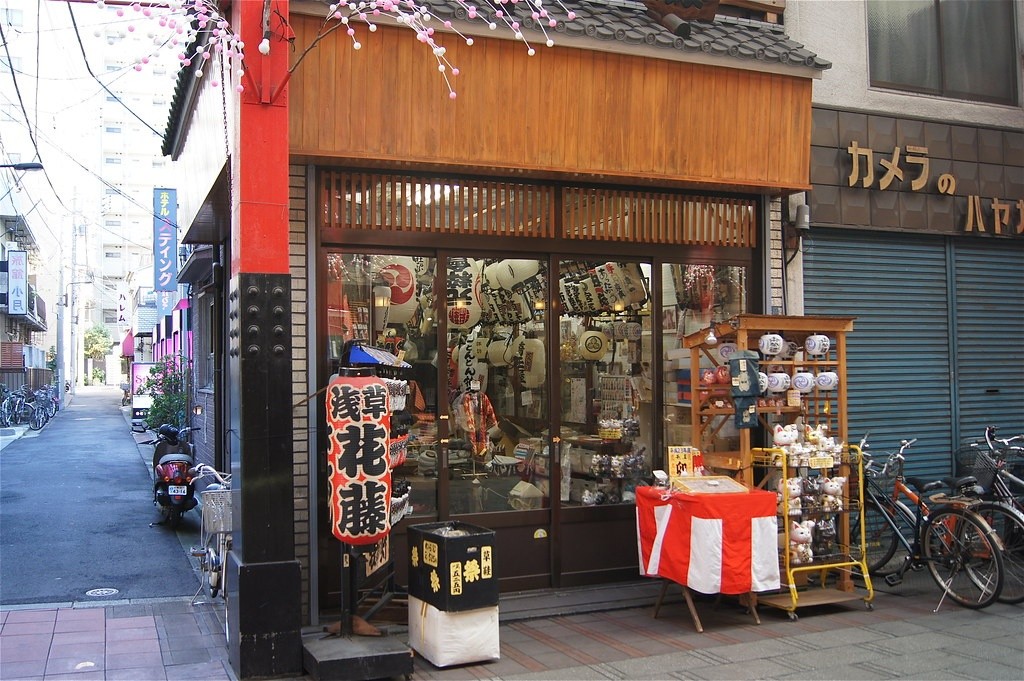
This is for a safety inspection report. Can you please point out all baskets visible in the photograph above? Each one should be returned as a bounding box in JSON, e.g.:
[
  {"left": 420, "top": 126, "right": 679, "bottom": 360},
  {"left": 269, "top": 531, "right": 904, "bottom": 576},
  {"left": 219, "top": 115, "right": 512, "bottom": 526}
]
[
  {"left": 954, "top": 445, "right": 998, "bottom": 490},
  {"left": 201, "top": 489, "right": 234, "bottom": 535},
  {"left": 866, "top": 451, "right": 899, "bottom": 488}
]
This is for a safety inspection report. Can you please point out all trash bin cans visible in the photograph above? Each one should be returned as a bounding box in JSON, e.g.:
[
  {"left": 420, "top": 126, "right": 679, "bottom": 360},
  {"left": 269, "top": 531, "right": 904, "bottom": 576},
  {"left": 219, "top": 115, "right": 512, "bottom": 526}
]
[
  {"left": 406, "top": 520, "right": 501, "bottom": 668},
  {"left": 510, "top": 480, "right": 543, "bottom": 509}
]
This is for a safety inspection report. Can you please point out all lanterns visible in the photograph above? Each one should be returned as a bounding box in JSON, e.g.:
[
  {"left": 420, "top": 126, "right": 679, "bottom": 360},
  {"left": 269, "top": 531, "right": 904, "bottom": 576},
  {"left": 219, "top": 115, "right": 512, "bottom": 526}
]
[
  {"left": 717, "top": 334, "right": 838, "bottom": 392},
  {"left": 325, "top": 370, "right": 392, "bottom": 544},
  {"left": 371, "top": 253, "right": 647, "bottom": 392}
]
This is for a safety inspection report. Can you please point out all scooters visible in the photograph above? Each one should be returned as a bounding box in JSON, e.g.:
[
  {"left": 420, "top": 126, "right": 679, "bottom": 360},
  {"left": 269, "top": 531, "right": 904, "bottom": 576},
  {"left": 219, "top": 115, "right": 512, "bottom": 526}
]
[{"left": 137, "top": 423, "right": 203, "bottom": 527}]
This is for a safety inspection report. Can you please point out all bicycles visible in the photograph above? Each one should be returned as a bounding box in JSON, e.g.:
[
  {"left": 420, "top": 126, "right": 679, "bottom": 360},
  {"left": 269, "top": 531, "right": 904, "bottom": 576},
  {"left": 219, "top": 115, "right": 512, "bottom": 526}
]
[
  {"left": 957, "top": 423, "right": 1024, "bottom": 511},
  {"left": 186, "top": 461, "right": 234, "bottom": 650},
  {"left": 0, "top": 380, "right": 59, "bottom": 434},
  {"left": 835, "top": 437, "right": 1024, "bottom": 604},
  {"left": 853, "top": 428, "right": 1006, "bottom": 616}
]
